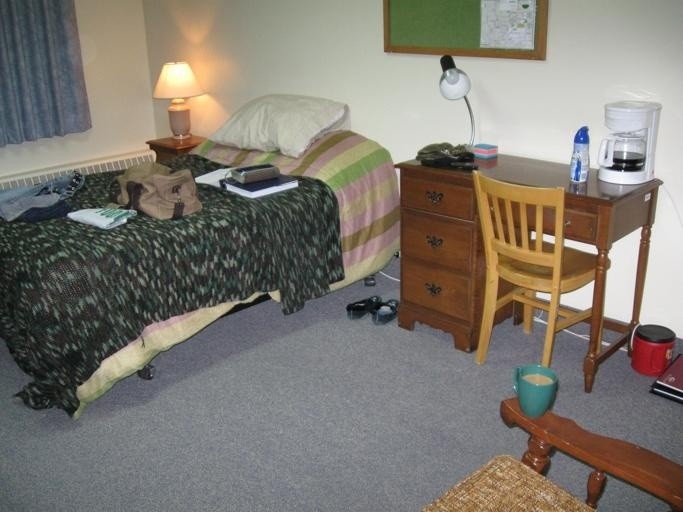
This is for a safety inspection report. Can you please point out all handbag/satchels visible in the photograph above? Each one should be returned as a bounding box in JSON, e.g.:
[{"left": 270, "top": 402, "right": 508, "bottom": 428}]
[{"left": 115, "top": 162, "right": 202, "bottom": 221}]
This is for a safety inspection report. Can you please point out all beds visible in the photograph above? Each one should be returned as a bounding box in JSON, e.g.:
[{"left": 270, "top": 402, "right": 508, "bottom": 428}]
[{"left": 0, "top": 128, "right": 405, "bottom": 422}]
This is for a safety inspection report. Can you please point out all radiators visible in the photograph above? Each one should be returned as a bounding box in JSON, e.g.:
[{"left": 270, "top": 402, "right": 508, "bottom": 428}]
[{"left": 0, "top": 151, "right": 155, "bottom": 192}]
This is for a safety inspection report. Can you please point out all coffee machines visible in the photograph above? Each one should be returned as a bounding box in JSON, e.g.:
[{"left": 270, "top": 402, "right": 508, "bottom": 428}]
[
  {"left": 596, "top": 99, "right": 661, "bottom": 184},
  {"left": 596, "top": 181, "right": 644, "bottom": 201}
]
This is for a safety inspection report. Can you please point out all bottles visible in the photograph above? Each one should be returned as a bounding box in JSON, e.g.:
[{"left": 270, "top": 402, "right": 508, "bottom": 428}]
[
  {"left": 567, "top": 181, "right": 587, "bottom": 194},
  {"left": 567, "top": 127, "right": 590, "bottom": 184}
]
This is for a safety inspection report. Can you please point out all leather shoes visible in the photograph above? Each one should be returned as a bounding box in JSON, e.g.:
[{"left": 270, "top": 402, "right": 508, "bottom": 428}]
[
  {"left": 373, "top": 300, "right": 398, "bottom": 324},
  {"left": 346, "top": 296, "right": 380, "bottom": 320}
]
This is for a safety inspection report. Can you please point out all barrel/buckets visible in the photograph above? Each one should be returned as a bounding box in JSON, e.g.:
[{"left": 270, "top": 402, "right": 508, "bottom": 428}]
[{"left": 631, "top": 325, "right": 675, "bottom": 377}]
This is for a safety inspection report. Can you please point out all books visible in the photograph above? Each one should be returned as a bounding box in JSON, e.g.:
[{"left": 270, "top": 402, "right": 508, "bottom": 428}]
[
  {"left": 219, "top": 177, "right": 298, "bottom": 199},
  {"left": 650, "top": 354, "right": 683, "bottom": 403}
]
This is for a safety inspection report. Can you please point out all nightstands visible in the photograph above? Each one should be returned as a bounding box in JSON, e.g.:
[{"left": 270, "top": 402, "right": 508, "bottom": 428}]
[{"left": 146, "top": 137, "right": 206, "bottom": 162}]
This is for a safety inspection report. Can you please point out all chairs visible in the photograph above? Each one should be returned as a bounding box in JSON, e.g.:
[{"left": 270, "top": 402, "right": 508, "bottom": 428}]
[
  {"left": 418, "top": 396, "right": 683, "bottom": 512},
  {"left": 473, "top": 170, "right": 610, "bottom": 364}
]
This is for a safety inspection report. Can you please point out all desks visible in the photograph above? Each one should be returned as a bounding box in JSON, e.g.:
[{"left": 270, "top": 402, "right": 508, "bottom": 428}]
[{"left": 394, "top": 151, "right": 661, "bottom": 394}]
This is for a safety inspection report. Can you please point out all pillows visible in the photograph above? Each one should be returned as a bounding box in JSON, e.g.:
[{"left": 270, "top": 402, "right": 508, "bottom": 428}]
[{"left": 208, "top": 94, "right": 347, "bottom": 159}]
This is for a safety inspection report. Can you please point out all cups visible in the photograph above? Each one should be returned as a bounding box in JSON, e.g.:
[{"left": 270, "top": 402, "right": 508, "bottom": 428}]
[{"left": 510, "top": 364, "right": 557, "bottom": 418}]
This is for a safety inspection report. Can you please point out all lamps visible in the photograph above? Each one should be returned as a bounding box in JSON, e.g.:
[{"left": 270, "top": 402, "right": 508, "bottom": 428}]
[
  {"left": 439, "top": 53, "right": 476, "bottom": 159},
  {"left": 153, "top": 63, "right": 206, "bottom": 136}
]
